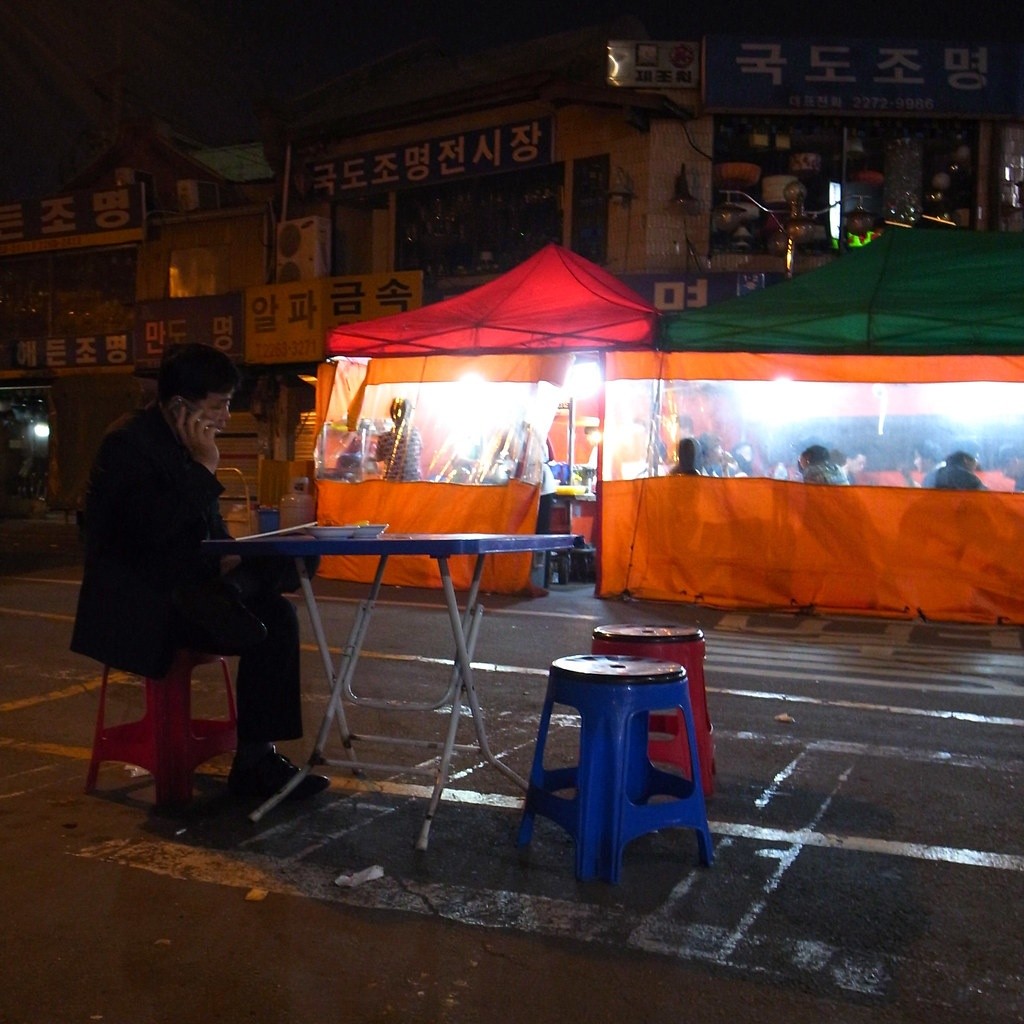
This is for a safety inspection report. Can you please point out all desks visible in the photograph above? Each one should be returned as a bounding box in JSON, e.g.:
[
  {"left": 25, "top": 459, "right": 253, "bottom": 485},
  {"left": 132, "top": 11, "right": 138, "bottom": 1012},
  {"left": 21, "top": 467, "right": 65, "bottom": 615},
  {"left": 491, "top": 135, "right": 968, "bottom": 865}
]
[
  {"left": 201, "top": 534, "right": 585, "bottom": 853},
  {"left": 536, "top": 492, "right": 598, "bottom": 585}
]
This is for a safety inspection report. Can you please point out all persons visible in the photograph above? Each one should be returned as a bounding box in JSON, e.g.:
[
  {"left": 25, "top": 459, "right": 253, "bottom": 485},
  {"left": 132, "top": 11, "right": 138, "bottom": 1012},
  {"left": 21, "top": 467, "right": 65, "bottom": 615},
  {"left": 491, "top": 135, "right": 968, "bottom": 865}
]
[
  {"left": 79, "top": 342, "right": 328, "bottom": 801},
  {"left": 341, "top": 398, "right": 1024, "bottom": 490}
]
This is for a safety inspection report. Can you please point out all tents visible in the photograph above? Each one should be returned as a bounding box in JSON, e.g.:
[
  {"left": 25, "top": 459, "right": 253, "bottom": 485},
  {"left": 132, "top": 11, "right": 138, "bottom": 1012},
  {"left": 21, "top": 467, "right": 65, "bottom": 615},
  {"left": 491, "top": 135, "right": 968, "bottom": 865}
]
[
  {"left": 597, "top": 224, "right": 1024, "bottom": 630},
  {"left": 324, "top": 242, "right": 662, "bottom": 598}
]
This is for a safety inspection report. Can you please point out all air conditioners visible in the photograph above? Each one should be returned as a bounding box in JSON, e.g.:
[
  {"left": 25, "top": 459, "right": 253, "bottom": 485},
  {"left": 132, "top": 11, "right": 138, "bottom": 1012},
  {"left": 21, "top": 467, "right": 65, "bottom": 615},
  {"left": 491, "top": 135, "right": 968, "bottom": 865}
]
[
  {"left": 275, "top": 216, "right": 331, "bottom": 282},
  {"left": 177, "top": 178, "right": 220, "bottom": 213}
]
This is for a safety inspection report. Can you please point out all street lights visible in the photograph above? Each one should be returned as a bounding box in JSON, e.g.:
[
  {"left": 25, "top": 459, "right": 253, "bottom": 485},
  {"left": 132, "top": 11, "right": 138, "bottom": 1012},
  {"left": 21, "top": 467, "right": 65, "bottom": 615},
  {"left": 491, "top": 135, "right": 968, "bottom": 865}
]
[{"left": 713, "top": 179, "right": 876, "bottom": 281}]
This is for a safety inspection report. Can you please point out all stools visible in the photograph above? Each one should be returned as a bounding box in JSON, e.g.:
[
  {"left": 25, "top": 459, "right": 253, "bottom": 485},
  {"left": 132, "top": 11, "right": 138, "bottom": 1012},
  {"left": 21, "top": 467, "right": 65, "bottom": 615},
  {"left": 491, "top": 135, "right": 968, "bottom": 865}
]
[
  {"left": 517, "top": 655, "right": 715, "bottom": 886},
  {"left": 88, "top": 656, "right": 236, "bottom": 816},
  {"left": 590, "top": 624, "right": 716, "bottom": 796}
]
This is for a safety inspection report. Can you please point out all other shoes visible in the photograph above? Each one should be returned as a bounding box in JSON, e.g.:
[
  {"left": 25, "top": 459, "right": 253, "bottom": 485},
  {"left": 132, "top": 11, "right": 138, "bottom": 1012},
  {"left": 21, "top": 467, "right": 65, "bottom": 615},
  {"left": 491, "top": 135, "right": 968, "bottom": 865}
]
[{"left": 226, "top": 745, "right": 331, "bottom": 805}]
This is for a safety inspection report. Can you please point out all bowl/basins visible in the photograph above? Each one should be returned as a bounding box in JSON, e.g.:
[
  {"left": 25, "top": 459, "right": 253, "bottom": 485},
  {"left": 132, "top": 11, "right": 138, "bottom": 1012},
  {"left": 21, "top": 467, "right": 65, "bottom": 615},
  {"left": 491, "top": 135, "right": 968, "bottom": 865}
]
[
  {"left": 345, "top": 523, "right": 389, "bottom": 538},
  {"left": 303, "top": 525, "right": 360, "bottom": 539}
]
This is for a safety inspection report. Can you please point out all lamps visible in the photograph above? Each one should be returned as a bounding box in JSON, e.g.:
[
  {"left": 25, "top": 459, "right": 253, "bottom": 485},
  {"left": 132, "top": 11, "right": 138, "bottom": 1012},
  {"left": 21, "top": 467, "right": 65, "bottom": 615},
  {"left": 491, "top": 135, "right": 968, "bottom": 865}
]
[
  {"left": 605, "top": 166, "right": 632, "bottom": 203},
  {"left": 668, "top": 162, "right": 699, "bottom": 205}
]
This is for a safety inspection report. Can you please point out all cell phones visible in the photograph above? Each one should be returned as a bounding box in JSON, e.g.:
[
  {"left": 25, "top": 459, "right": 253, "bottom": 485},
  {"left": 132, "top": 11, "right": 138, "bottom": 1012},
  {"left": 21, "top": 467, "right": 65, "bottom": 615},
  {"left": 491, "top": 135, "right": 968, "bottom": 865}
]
[{"left": 169, "top": 396, "right": 208, "bottom": 433}]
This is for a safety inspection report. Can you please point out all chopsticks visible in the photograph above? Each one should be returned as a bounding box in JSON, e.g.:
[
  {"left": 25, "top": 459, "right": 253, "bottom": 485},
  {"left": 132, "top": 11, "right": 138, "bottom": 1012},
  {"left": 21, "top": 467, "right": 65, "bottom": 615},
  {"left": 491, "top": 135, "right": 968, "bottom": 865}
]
[{"left": 235, "top": 521, "right": 318, "bottom": 540}]
